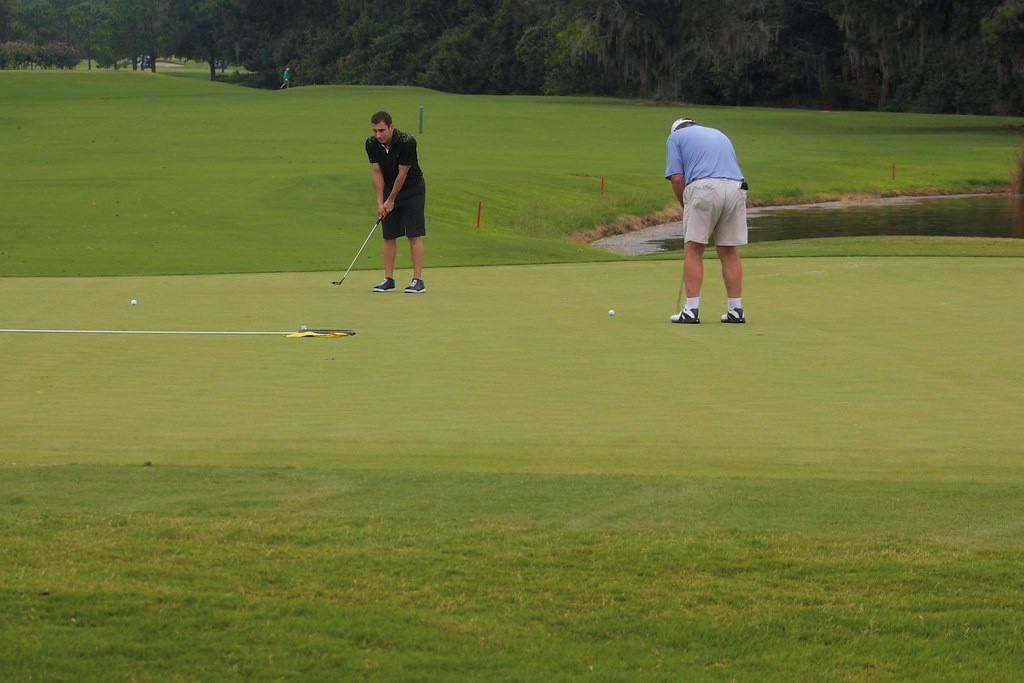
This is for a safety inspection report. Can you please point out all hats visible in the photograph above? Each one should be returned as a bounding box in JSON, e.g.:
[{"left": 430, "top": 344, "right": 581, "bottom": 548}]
[
  {"left": 670, "top": 118, "right": 696, "bottom": 134},
  {"left": 286, "top": 68, "right": 289, "bottom": 70}
]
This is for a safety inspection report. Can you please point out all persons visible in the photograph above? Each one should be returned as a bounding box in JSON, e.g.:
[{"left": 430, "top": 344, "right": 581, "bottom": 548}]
[
  {"left": 280, "top": 68, "right": 290, "bottom": 88},
  {"left": 139, "top": 54, "right": 151, "bottom": 66},
  {"left": 365, "top": 111, "right": 426, "bottom": 293},
  {"left": 664, "top": 116, "right": 748, "bottom": 324}
]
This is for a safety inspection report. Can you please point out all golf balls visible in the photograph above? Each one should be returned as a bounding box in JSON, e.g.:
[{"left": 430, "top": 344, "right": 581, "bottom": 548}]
[
  {"left": 609, "top": 309, "right": 615, "bottom": 316},
  {"left": 131, "top": 299, "right": 137, "bottom": 305}
]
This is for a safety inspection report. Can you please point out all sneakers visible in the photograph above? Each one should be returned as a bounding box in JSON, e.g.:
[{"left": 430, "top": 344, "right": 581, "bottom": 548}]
[
  {"left": 721, "top": 308, "right": 745, "bottom": 323},
  {"left": 670, "top": 307, "right": 700, "bottom": 323},
  {"left": 372, "top": 276, "right": 395, "bottom": 292},
  {"left": 403, "top": 277, "right": 426, "bottom": 293}
]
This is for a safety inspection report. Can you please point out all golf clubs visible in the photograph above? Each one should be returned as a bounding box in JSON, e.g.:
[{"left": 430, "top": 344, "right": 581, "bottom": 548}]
[
  {"left": 330, "top": 216, "right": 383, "bottom": 286},
  {"left": 676, "top": 277, "right": 684, "bottom": 313}
]
